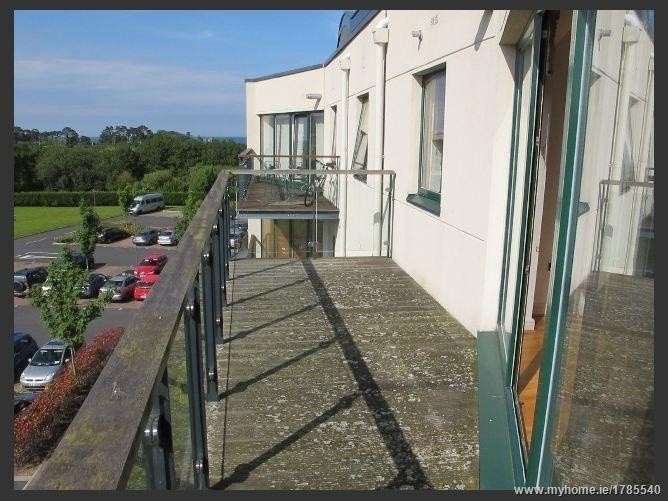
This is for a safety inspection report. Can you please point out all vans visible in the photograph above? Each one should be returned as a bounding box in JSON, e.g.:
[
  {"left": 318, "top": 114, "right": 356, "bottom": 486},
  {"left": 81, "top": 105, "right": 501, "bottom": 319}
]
[
  {"left": 14, "top": 332, "right": 39, "bottom": 379},
  {"left": 127, "top": 193, "right": 165, "bottom": 216}
]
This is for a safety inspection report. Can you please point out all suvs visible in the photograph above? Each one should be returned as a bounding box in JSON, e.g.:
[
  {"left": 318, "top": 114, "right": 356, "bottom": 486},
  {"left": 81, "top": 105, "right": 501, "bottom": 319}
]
[{"left": 14, "top": 265, "right": 47, "bottom": 298}]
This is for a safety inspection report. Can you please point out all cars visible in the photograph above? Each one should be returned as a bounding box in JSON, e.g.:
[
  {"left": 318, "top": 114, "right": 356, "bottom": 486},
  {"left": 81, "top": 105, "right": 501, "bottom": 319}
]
[
  {"left": 134, "top": 274, "right": 159, "bottom": 302},
  {"left": 72, "top": 273, "right": 108, "bottom": 298},
  {"left": 131, "top": 228, "right": 161, "bottom": 246},
  {"left": 156, "top": 229, "right": 178, "bottom": 245},
  {"left": 19, "top": 335, "right": 88, "bottom": 390},
  {"left": 40, "top": 277, "right": 58, "bottom": 300},
  {"left": 229, "top": 228, "right": 244, "bottom": 249},
  {"left": 97, "top": 272, "right": 138, "bottom": 302},
  {"left": 133, "top": 253, "right": 168, "bottom": 276},
  {"left": 13, "top": 391, "right": 40, "bottom": 417},
  {"left": 66, "top": 252, "right": 95, "bottom": 270},
  {"left": 95, "top": 226, "right": 127, "bottom": 245}
]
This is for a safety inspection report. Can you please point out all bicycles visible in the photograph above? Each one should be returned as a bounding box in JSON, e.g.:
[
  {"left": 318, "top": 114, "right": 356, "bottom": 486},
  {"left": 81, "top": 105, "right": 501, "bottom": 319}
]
[{"left": 303, "top": 158, "right": 335, "bottom": 207}]
